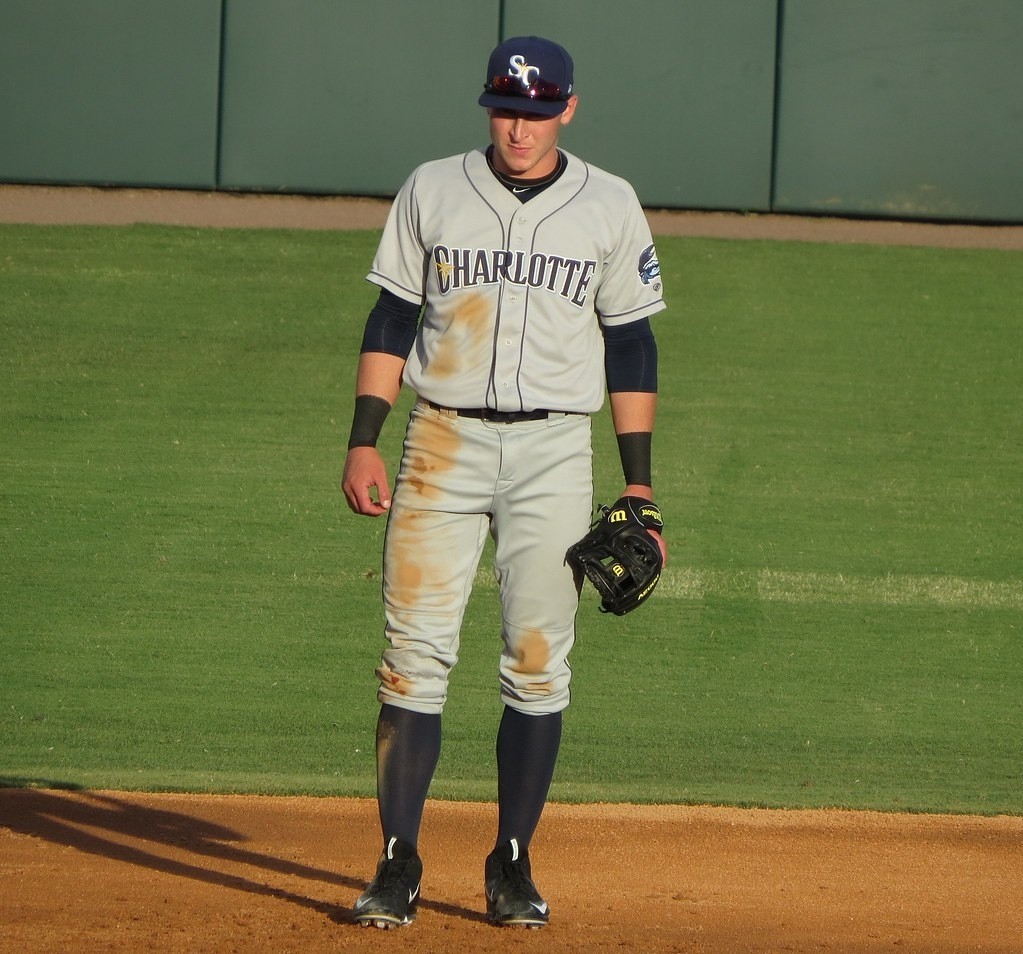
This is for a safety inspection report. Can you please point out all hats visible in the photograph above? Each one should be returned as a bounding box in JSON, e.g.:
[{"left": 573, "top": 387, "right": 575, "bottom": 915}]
[{"left": 477, "top": 35, "right": 574, "bottom": 115}]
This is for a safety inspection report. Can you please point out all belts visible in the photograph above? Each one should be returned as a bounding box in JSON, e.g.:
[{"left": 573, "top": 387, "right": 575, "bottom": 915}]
[{"left": 429, "top": 400, "right": 567, "bottom": 422}]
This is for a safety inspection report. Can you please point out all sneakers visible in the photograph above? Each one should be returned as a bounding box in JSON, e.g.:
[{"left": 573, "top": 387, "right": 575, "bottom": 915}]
[
  {"left": 351, "top": 838, "right": 422, "bottom": 929},
  {"left": 485, "top": 839, "right": 548, "bottom": 931}
]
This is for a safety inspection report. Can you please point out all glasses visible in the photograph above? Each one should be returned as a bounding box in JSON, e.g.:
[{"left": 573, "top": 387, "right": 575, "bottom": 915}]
[{"left": 483, "top": 76, "right": 571, "bottom": 101}]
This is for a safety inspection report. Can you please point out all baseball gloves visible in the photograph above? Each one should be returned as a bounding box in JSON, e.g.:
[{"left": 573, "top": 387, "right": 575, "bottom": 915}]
[{"left": 565, "top": 496, "right": 664, "bottom": 617}]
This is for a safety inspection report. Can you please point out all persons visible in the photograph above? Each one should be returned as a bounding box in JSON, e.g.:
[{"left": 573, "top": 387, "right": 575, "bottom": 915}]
[{"left": 344, "top": 37, "right": 667, "bottom": 927}]
